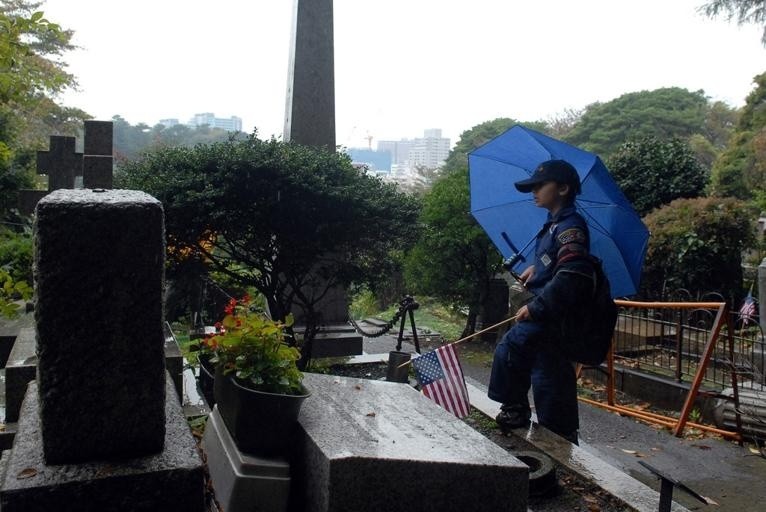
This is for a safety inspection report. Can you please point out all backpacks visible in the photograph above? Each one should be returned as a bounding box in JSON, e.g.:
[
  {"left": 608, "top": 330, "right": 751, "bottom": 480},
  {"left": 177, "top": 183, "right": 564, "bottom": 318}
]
[{"left": 550, "top": 253, "right": 617, "bottom": 366}]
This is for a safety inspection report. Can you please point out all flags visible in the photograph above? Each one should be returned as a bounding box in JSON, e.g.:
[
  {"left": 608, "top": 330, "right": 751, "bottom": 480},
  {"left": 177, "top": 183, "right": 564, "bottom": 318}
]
[
  {"left": 411, "top": 342, "right": 471, "bottom": 420},
  {"left": 739, "top": 288, "right": 756, "bottom": 324}
]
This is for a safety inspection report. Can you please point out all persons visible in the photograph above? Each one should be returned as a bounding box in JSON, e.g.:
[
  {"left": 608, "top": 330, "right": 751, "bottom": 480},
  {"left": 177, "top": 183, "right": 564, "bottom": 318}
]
[{"left": 488, "top": 160, "right": 603, "bottom": 453}]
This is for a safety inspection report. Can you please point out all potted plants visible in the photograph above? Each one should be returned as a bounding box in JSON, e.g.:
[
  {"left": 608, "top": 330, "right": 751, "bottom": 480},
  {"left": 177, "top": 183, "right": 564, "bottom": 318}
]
[{"left": 215, "top": 316, "right": 312, "bottom": 447}]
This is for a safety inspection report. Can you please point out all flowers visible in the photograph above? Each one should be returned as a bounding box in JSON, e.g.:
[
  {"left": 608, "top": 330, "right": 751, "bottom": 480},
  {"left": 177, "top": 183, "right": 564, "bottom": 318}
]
[{"left": 199, "top": 295, "right": 264, "bottom": 357}]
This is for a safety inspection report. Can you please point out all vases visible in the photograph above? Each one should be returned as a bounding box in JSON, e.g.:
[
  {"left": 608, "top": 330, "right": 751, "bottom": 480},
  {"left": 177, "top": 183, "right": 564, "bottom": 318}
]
[{"left": 198, "top": 355, "right": 215, "bottom": 405}]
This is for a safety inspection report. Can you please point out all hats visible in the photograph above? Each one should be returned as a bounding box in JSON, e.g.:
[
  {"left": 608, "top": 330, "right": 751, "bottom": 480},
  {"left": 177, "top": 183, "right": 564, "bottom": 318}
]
[{"left": 514, "top": 159, "right": 580, "bottom": 193}]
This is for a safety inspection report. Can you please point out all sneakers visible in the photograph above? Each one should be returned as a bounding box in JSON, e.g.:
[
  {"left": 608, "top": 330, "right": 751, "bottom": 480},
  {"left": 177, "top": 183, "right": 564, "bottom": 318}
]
[{"left": 496, "top": 402, "right": 531, "bottom": 429}]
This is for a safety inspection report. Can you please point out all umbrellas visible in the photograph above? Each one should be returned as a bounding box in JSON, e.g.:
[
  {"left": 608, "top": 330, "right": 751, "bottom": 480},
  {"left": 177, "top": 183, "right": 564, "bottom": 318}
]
[{"left": 469, "top": 123, "right": 653, "bottom": 299}]
